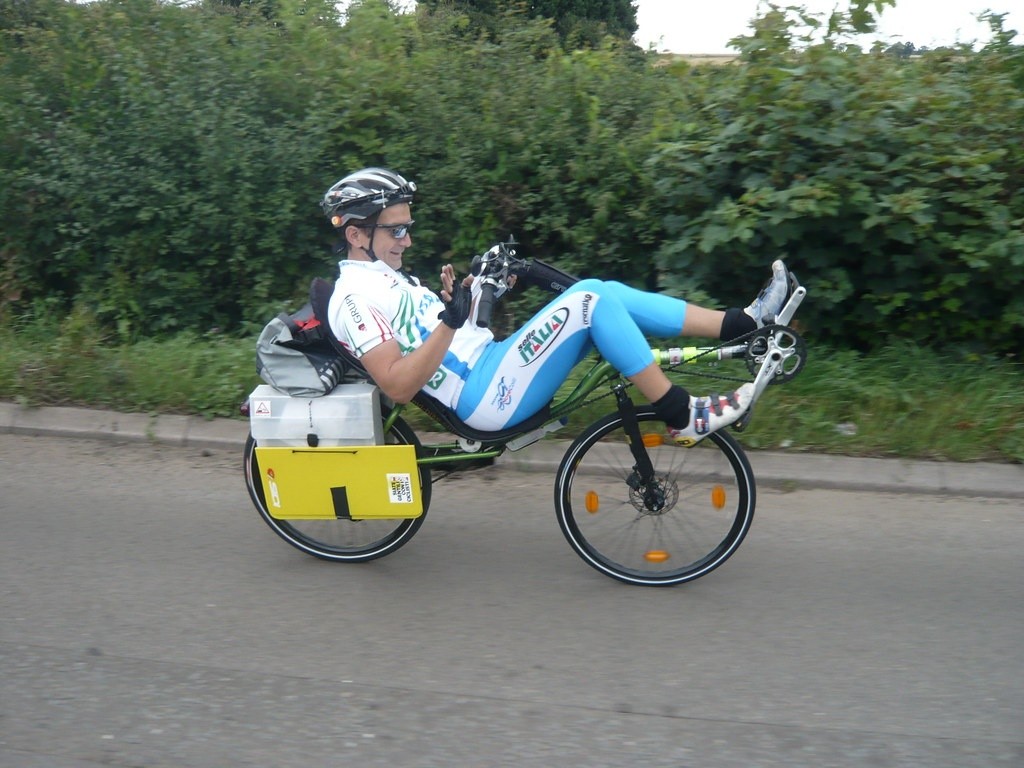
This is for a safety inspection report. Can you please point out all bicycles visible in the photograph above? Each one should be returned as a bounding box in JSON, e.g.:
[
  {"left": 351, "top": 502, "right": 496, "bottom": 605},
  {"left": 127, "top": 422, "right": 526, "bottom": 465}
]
[{"left": 241, "top": 236, "right": 807, "bottom": 587}]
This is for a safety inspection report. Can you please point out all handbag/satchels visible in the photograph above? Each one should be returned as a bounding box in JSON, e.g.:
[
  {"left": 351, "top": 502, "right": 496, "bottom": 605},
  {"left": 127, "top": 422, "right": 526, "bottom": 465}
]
[{"left": 255, "top": 306, "right": 350, "bottom": 398}]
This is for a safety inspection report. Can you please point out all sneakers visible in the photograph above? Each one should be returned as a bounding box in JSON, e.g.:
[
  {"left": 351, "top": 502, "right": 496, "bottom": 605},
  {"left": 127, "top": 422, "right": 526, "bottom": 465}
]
[
  {"left": 744, "top": 260, "right": 792, "bottom": 329},
  {"left": 668, "top": 382, "right": 754, "bottom": 448}
]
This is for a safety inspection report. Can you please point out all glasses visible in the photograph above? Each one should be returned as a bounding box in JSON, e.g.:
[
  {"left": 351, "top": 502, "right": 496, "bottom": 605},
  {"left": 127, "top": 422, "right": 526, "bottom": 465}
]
[{"left": 355, "top": 220, "right": 415, "bottom": 239}]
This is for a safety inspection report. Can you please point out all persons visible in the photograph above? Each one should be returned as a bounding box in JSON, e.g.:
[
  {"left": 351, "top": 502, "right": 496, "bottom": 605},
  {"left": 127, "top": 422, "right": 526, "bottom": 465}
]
[{"left": 324, "top": 168, "right": 793, "bottom": 447}]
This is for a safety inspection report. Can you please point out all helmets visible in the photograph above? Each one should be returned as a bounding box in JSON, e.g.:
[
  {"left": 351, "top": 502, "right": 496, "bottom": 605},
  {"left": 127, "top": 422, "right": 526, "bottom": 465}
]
[{"left": 320, "top": 167, "right": 417, "bottom": 229}]
